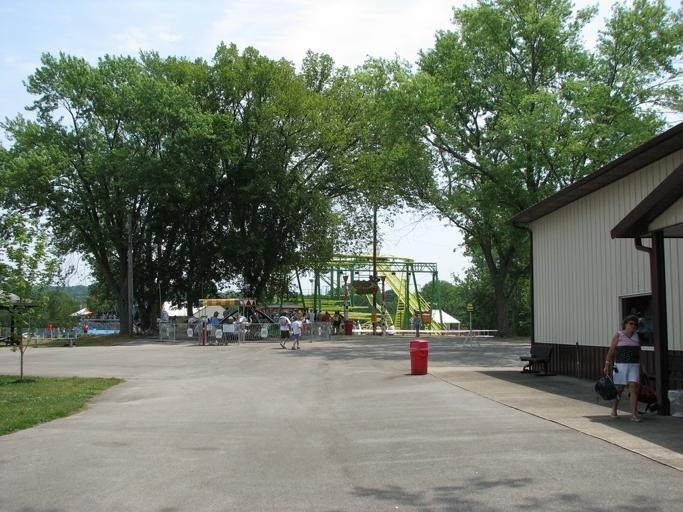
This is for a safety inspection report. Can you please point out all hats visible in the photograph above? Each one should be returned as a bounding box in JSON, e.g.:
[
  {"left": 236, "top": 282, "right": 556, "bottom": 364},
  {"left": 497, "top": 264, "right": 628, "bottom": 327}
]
[{"left": 214, "top": 311, "right": 219, "bottom": 314}]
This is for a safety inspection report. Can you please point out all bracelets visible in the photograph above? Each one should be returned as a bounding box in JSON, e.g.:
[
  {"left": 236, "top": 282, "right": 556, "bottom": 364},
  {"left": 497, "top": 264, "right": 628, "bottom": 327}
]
[{"left": 605, "top": 359, "right": 611, "bottom": 364}]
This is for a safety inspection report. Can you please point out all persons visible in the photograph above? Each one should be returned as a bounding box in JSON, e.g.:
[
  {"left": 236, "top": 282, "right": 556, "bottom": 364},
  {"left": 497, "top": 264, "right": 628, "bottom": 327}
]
[
  {"left": 288, "top": 308, "right": 345, "bottom": 335},
  {"left": 421, "top": 310, "right": 429, "bottom": 323},
  {"left": 291, "top": 315, "right": 302, "bottom": 350},
  {"left": 82, "top": 322, "right": 89, "bottom": 333},
  {"left": 355, "top": 320, "right": 358, "bottom": 325},
  {"left": 602, "top": 314, "right": 648, "bottom": 424},
  {"left": 269, "top": 314, "right": 276, "bottom": 323},
  {"left": 187, "top": 307, "right": 263, "bottom": 345},
  {"left": 379, "top": 312, "right": 394, "bottom": 337},
  {"left": 47, "top": 321, "right": 54, "bottom": 337},
  {"left": 278, "top": 312, "right": 292, "bottom": 349},
  {"left": 412, "top": 313, "right": 420, "bottom": 337}
]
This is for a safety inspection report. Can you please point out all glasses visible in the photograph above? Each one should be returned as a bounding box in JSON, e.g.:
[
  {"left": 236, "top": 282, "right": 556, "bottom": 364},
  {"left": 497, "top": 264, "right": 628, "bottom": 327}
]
[{"left": 629, "top": 322, "right": 638, "bottom": 326}]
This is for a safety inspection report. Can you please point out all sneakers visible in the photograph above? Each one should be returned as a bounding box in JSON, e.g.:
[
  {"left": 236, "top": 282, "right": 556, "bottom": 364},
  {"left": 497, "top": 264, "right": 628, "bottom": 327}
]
[
  {"left": 292, "top": 346, "right": 301, "bottom": 350},
  {"left": 611, "top": 411, "right": 618, "bottom": 417},
  {"left": 280, "top": 342, "right": 286, "bottom": 348},
  {"left": 631, "top": 415, "right": 642, "bottom": 422}
]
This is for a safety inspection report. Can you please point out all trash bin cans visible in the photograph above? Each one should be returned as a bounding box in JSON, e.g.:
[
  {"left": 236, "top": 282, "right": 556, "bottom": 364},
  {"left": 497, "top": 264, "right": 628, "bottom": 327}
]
[
  {"left": 409, "top": 339, "right": 429, "bottom": 375},
  {"left": 344, "top": 320, "right": 353, "bottom": 335}
]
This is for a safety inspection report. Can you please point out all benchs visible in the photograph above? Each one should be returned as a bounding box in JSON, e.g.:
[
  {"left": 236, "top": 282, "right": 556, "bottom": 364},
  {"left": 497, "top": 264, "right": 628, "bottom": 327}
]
[
  {"left": 519, "top": 345, "right": 557, "bottom": 377},
  {"left": 32, "top": 337, "right": 76, "bottom": 347}
]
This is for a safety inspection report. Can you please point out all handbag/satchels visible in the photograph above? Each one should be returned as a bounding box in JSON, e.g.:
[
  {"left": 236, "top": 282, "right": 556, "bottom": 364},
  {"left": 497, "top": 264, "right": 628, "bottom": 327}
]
[
  {"left": 594, "top": 374, "right": 617, "bottom": 400},
  {"left": 628, "top": 374, "right": 657, "bottom": 404}
]
